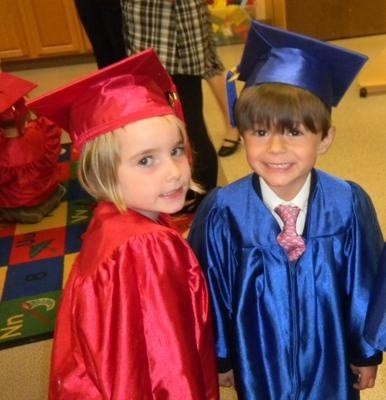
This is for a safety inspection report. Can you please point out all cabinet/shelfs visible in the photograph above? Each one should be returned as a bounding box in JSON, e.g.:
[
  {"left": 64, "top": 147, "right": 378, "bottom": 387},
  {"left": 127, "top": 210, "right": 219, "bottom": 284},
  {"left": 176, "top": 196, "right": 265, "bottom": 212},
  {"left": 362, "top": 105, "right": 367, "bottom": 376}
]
[{"left": 0, "top": 0, "right": 93, "bottom": 65}]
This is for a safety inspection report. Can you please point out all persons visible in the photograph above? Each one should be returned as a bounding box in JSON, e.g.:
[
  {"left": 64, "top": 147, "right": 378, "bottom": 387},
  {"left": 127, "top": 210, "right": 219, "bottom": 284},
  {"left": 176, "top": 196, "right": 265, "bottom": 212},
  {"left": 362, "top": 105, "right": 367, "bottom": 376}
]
[
  {"left": 202, "top": 19, "right": 242, "bottom": 157},
  {"left": 0, "top": 71, "right": 66, "bottom": 225},
  {"left": 185, "top": 20, "right": 385, "bottom": 400},
  {"left": 22, "top": 48, "right": 220, "bottom": 400},
  {"left": 121, "top": 0, "right": 223, "bottom": 214}
]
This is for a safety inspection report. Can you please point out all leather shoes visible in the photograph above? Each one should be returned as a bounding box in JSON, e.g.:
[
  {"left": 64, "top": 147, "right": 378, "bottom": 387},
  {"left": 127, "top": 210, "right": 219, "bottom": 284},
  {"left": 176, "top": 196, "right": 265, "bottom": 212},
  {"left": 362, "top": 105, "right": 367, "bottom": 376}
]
[{"left": 218, "top": 138, "right": 240, "bottom": 157}]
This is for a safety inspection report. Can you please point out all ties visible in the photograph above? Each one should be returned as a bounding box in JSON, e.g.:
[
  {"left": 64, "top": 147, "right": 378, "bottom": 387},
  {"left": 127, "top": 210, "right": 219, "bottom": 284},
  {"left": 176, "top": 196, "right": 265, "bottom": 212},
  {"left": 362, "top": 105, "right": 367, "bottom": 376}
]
[{"left": 273, "top": 204, "right": 305, "bottom": 262}]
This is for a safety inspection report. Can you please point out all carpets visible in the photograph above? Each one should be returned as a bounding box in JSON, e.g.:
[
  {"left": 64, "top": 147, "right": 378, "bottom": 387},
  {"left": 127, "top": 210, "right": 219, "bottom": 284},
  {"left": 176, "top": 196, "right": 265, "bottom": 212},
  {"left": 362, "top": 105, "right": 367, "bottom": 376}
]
[{"left": 0, "top": 140, "right": 197, "bottom": 350}]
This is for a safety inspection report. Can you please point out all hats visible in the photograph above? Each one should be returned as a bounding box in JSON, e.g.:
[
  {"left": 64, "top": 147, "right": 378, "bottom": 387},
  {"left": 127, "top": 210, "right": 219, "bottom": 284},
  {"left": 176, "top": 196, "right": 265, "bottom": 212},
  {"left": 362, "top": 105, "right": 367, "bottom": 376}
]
[
  {"left": 225, "top": 19, "right": 369, "bottom": 127},
  {"left": 0, "top": 70, "right": 36, "bottom": 136},
  {"left": 25, "top": 47, "right": 195, "bottom": 161}
]
